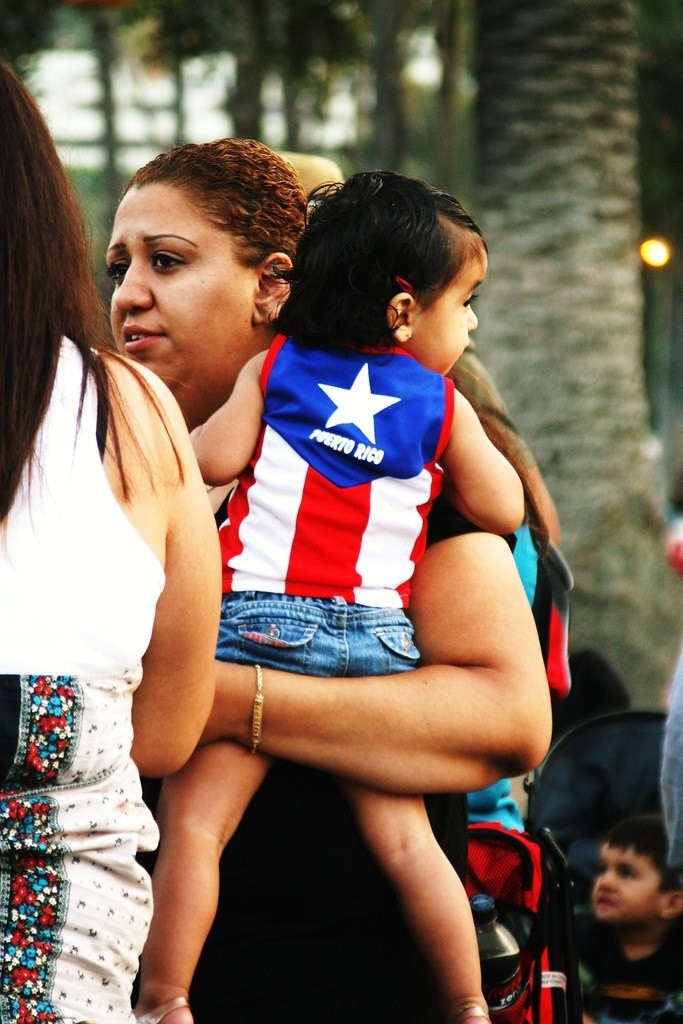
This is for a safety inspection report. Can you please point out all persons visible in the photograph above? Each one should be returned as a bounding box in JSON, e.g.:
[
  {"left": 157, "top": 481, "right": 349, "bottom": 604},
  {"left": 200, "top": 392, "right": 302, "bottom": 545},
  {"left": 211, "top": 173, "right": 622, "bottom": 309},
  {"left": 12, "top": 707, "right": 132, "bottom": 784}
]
[
  {"left": 581, "top": 811, "right": 683, "bottom": 1023},
  {"left": 106, "top": 140, "right": 562, "bottom": 1024},
  {"left": 128, "top": 169, "right": 525, "bottom": 1024},
  {"left": 0, "top": 63, "right": 223, "bottom": 1024}
]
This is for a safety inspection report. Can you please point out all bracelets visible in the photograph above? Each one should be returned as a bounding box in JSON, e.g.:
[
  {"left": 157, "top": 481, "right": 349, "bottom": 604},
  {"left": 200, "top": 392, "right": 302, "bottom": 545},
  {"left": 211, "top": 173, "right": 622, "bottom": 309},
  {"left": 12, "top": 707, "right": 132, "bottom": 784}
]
[{"left": 251, "top": 665, "right": 262, "bottom": 752}]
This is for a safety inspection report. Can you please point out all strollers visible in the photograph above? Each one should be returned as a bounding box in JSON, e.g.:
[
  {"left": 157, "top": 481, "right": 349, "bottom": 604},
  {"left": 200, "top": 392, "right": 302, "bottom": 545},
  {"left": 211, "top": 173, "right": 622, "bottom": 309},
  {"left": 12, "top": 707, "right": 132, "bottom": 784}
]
[
  {"left": 523, "top": 709, "right": 668, "bottom": 937},
  {"left": 466, "top": 821, "right": 586, "bottom": 1024}
]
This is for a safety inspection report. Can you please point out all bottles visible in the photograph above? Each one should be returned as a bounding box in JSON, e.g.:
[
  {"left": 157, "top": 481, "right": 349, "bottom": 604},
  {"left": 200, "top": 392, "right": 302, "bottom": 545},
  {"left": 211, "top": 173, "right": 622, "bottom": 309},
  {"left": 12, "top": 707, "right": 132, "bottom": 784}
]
[{"left": 470, "top": 893, "right": 523, "bottom": 1012}]
